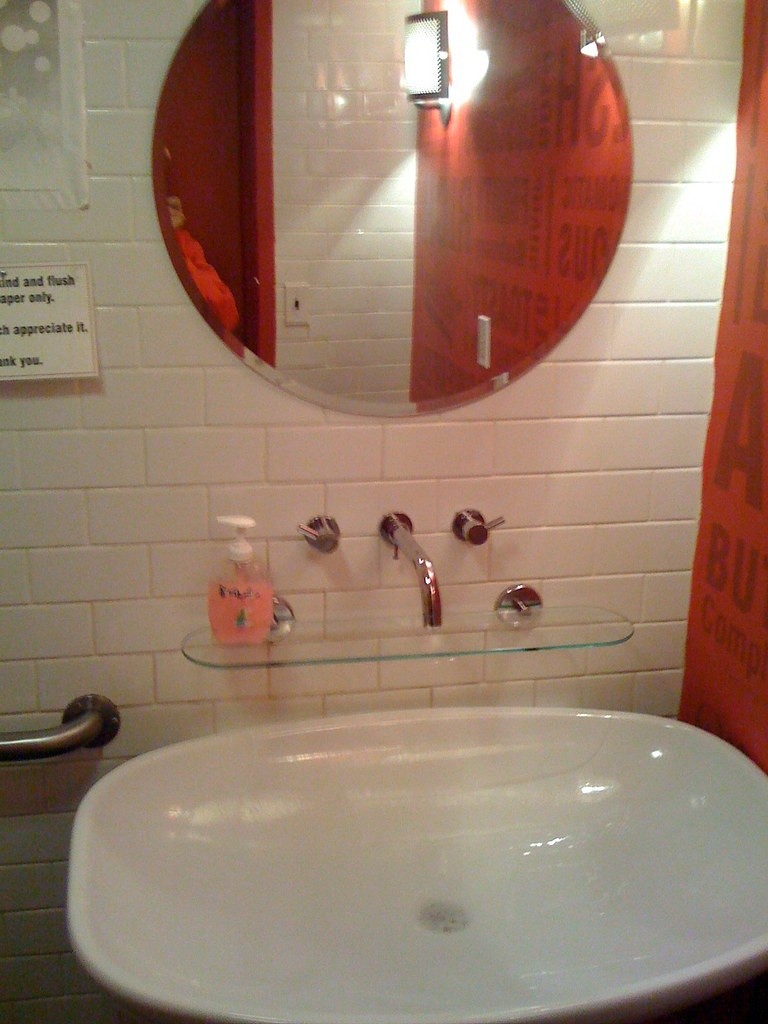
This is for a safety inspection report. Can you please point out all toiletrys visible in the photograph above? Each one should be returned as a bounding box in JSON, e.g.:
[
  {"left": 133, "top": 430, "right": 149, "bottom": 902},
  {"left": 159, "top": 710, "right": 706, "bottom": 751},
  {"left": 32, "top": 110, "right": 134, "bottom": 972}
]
[{"left": 207, "top": 513, "right": 274, "bottom": 646}]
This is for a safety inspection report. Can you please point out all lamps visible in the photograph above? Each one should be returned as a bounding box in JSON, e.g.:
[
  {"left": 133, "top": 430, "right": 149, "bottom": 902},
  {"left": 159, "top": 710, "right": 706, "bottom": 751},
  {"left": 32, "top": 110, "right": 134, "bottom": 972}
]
[{"left": 402, "top": 11, "right": 491, "bottom": 129}]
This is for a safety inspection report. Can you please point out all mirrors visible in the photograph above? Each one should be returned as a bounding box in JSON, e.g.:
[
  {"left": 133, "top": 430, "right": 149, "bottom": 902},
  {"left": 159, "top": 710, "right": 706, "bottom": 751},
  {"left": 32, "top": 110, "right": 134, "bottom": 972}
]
[{"left": 150, "top": 1, "right": 638, "bottom": 416}]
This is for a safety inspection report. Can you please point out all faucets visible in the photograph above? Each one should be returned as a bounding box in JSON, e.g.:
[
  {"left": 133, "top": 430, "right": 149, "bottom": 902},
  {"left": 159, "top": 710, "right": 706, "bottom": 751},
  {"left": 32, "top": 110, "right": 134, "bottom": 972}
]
[{"left": 387, "top": 520, "right": 444, "bottom": 630}]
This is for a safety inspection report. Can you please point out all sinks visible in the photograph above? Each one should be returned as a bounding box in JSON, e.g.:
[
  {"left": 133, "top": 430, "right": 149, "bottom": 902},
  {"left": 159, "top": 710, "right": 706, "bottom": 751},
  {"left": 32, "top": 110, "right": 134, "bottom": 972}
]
[{"left": 66, "top": 704, "right": 768, "bottom": 1024}]
